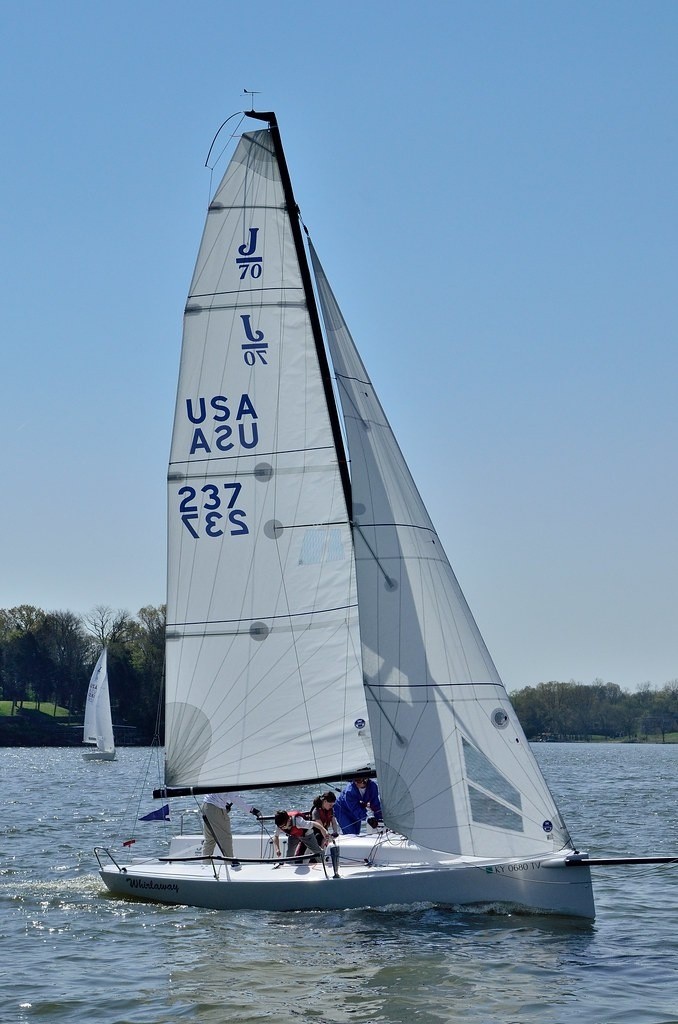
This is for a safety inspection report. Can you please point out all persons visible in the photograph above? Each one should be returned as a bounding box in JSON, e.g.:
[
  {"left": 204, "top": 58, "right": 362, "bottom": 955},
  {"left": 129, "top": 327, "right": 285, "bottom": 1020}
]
[
  {"left": 334, "top": 777, "right": 384, "bottom": 834},
  {"left": 202, "top": 791, "right": 263, "bottom": 867},
  {"left": 273, "top": 792, "right": 339, "bottom": 864}
]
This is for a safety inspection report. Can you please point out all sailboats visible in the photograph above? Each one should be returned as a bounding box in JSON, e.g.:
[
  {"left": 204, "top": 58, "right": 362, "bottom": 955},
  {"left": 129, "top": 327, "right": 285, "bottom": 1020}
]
[
  {"left": 95, "top": 88, "right": 597, "bottom": 922},
  {"left": 82, "top": 647, "right": 117, "bottom": 763}
]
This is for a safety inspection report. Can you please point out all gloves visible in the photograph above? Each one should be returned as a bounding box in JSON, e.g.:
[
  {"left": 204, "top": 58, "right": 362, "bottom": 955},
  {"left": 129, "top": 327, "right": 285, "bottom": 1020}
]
[
  {"left": 367, "top": 817, "right": 378, "bottom": 828},
  {"left": 250, "top": 808, "right": 262, "bottom": 818},
  {"left": 225, "top": 802, "right": 233, "bottom": 812},
  {"left": 331, "top": 832, "right": 338, "bottom": 838}
]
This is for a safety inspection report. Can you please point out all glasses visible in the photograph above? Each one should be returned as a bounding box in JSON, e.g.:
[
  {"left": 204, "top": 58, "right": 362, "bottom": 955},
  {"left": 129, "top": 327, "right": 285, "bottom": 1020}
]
[
  {"left": 279, "top": 820, "right": 287, "bottom": 829},
  {"left": 355, "top": 779, "right": 366, "bottom": 784}
]
